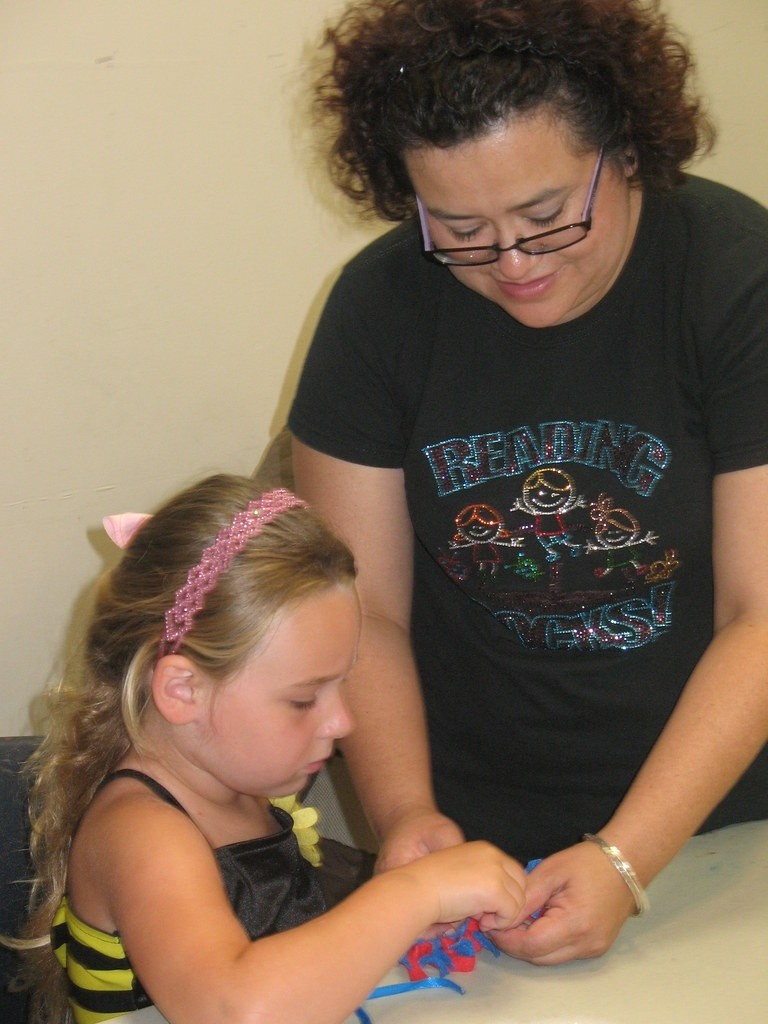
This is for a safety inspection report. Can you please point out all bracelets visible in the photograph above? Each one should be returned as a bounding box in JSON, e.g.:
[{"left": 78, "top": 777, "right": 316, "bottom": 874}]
[{"left": 582, "top": 832, "right": 652, "bottom": 921}]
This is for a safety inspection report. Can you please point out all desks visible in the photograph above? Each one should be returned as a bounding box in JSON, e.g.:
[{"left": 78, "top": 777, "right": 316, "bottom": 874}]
[{"left": 101, "top": 817, "right": 768, "bottom": 1022}]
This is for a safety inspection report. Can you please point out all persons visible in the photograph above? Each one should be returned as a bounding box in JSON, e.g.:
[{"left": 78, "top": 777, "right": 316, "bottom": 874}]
[
  {"left": 14, "top": 473, "right": 533, "bottom": 1024},
  {"left": 287, "top": 1, "right": 768, "bottom": 963}
]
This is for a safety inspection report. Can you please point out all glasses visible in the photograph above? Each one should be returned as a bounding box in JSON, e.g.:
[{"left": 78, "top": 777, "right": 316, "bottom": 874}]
[{"left": 413, "top": 142, "right": 606, "bottom": 267}]
